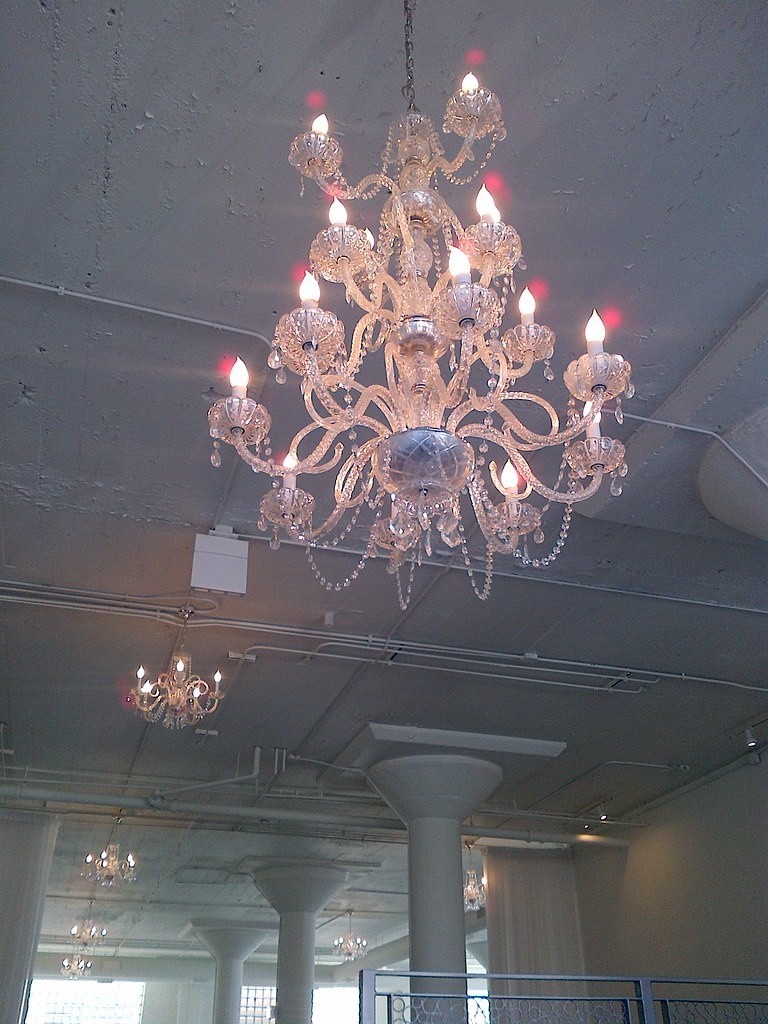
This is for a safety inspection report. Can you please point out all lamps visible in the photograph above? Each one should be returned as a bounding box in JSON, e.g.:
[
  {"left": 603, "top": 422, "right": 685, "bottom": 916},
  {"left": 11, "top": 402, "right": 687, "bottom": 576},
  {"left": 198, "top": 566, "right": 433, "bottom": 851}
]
[
  {"left": 208, "top": 0, "right": 636, "bottom": 613},
  {"left": 70, "top": 901, "right": 107, "bottom": 950},
  {"left": 334, "top": 909, "right": 367, "bottom": 961},
  {"left": 743, "top": 729, "right": 757, "bottom": 747},
  {"left": 596, "top": 806, "right": 607, "bottom": 820},
  {"left": 60, "top": 950, "right": 93, "bottom": 977},
  {"left": 464, "top": 842, "right": 487, "bottom": 912},
  {"left": 125, "top": 604, "right": 224, "bottom": 730},
  {"left": 80, "top": 815, "right": 137, "bottom": 889}
]
